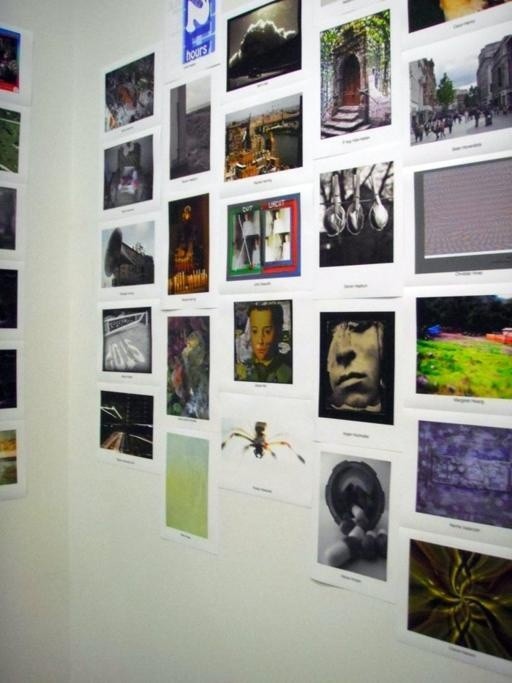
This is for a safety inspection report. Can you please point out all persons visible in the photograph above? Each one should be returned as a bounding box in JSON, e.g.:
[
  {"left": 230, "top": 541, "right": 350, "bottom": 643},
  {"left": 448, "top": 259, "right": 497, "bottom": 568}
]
[
  {"left": 232, "top": 302, "right": 291, "bottom": 385},
  {"left": 412, "top": 102, "right": 508, "bottom": 143},
  {"left": 327, "top": 317, "right": 387, "bottom": 414}
]
